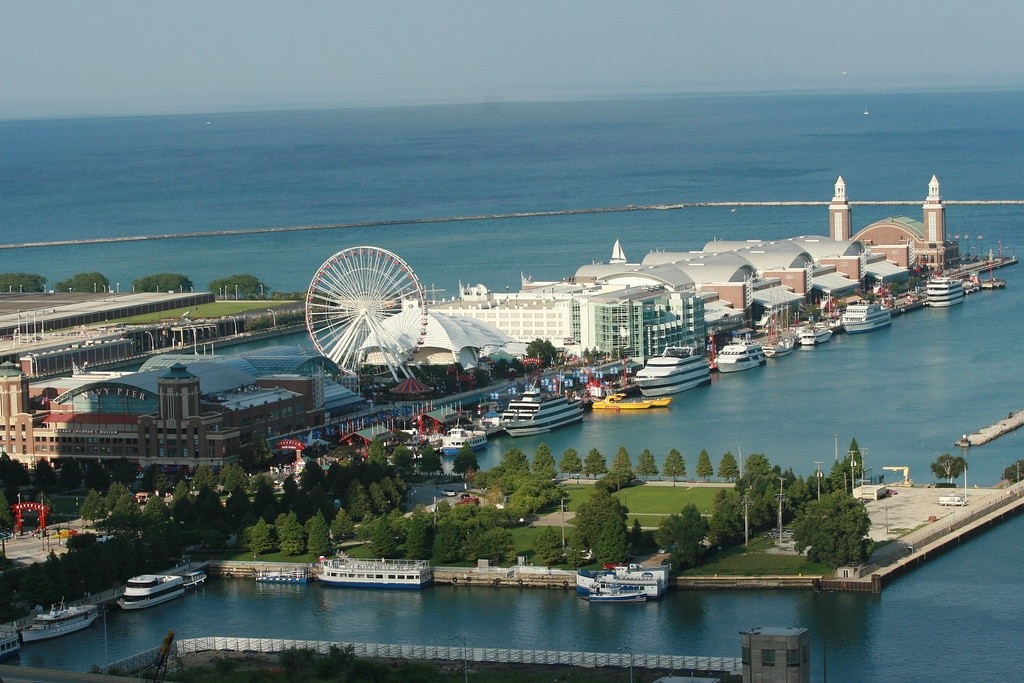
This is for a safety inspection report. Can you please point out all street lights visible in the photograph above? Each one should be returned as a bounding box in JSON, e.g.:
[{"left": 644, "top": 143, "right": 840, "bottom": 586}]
[
  {"left": 219, "top": 288, "right": 221, "bottom": 300},
  {"left": 235, "top": 285, "right": 238, "bottom": 301},
  {"left": 13, "top": 311, "right": 46, "bottom": 346},
  {"left": 9, "top": 282, "right": 194, "bottom": 293},
  {"left": 260, "top": 284, "right": 263, "bottom": 296},
  {"left": 225, "top": 286, "right": 228, "bottom": 301},
  {"left": 268, "top": 309, "right": 275, "bottom": 330}
]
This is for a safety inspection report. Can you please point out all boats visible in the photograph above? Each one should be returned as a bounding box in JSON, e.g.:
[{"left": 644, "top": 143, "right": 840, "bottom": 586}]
[
  {"left": 762, "top": 336, "right": 796, "bottom": 358},
  {"left": 500, "top": 388, "right": 585, "bottom": 436},
  {"left": 840, "top": 299, "right": 893, "bottom": 334},
  {"left": 714, "top": 339, "right": 767, "bottom": 372},
  {"left": 0, "top": 621, "right": 21, "bottom": 655},
  {"left": 21, "top": 596, "right": 98, "bottom": 642},
  {"left": 592, "top": 392, "right": 672, "bottom": 409},
  {"left": 576, "top": 566, "right": 670, "bottom": 603},
  {"left": 181, "top": 570, "right": 208, "bottom": 588},
  {"left": 254, "top": 568, "right": 310, "bottom": 585},
  {"left": 115, "top": 571, "right": 185, "bottom": 610},
  {"left": 442, "top": 425, "right": 488, "bottom": 456},
  {"left": 314, "top": 556, "right": 434, "bottom": 590},
  {"left": 926, "top": 274, "right": 964, "bottom": 306},
  {"left": 634, "top": 345, "right": 711, "bottom": 397},
  {"left": 794, "top": 324, "right": 833, "bottom": 345}
]
